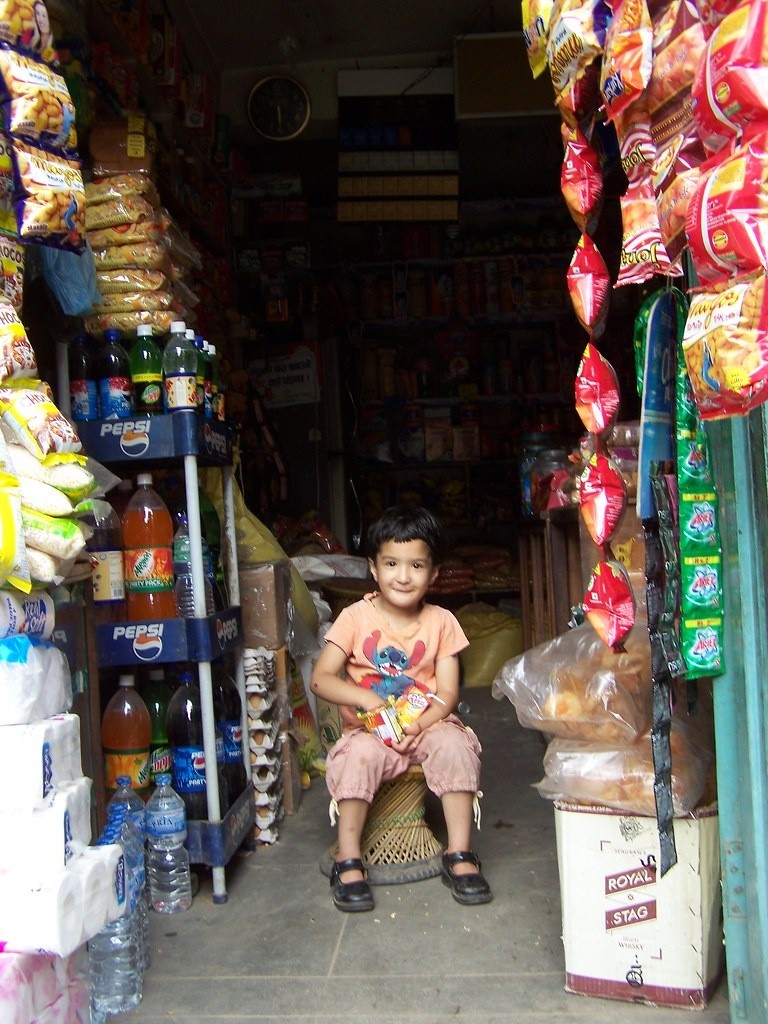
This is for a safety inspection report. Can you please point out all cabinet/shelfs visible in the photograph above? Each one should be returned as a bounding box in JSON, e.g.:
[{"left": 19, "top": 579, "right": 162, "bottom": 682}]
[
  {"left": 84, "top": 408, "right": 260, "bottom": 907},
  {"left": 313, "top": 195, "right": 577, "bottom": 559},
  {"left": 518, "top": 519, "right": 570, "bottom": 655}
]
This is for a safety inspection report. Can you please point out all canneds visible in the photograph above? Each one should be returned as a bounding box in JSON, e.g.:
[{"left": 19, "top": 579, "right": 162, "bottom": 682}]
[{"left": 519, "top": 443, "right": 576, "bottom": 516}]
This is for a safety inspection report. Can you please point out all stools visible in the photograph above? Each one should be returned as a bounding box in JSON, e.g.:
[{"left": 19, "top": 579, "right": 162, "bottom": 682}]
[{"left": 317, "top": 762, "right": 448, "bottom": 886}]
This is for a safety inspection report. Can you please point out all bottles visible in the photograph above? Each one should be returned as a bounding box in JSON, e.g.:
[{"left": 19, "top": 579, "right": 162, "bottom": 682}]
[
  {"left": 164, "top": 321, "right": 226, "bottom": 423},
  {"left": 72, "top": 320, "right": 99, "bottom": 422},
  {"left": 50, "top": 472, "right": 224, "bottom": 622},
  {"left": 129, "top": 325, "right": 163, "bottom": 416},
  {"left": 101, "top": 330, "right": 133, "bottom": 421},
  {"left": 146, "top": 773, "right": 192, "bottom": 913},
  {"left": 88, "top": 656, "right": 248, "bottom": 1014}
]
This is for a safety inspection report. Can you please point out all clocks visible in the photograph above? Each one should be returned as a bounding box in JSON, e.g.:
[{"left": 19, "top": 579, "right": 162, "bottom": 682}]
[{"left": 247, "top": 75, "right": 313, "bottom": 143}]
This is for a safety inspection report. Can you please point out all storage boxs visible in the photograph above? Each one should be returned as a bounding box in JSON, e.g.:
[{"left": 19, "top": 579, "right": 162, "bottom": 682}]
[
  {"left": 238, "top": 562, "right": 289, "bottom": 651},
  {"left": 272, "top": 645, "right": 292, "bottom": 731},
  {"left": 277, "top": 731, "right": 304, "bottom": 817},
  {"left": 552, "top": 788, "right": 721, "bottom": 1013}
]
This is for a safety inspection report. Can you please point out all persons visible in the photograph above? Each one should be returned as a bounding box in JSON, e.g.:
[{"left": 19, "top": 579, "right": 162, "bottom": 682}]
[{"left": 308, "top": 503, "right": 495, "bottom": 915}]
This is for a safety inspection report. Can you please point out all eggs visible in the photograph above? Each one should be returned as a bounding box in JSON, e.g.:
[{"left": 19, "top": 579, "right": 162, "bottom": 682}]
[{"left": 246, "top": 693, "right": 270, "bottom": 837}]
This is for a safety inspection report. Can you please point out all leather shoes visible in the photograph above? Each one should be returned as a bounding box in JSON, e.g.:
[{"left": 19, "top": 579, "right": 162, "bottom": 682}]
[
  {"left": 440, "top": 848, "right": 493, "bottom": 904},
  {"left": 330, "top": 858, "right": 374, "bottom": 910}
]
